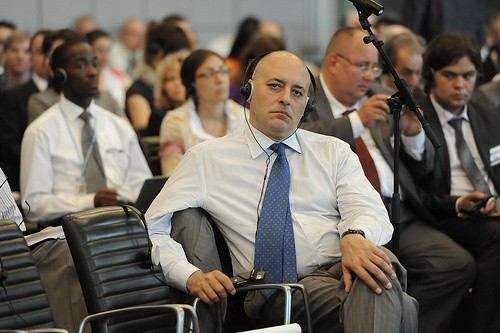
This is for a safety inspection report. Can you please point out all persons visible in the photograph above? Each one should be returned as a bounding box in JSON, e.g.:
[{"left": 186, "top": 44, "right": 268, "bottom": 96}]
[
  {"left": 159, "top": 49, "right": 250, "bottom": 177},
  {"left": 224, "top": 15, "right": 285, "bottom": 94},
  {"left": 301, "top": 27, "right": 476, "bottom": 333},
  {"left": 0, "top": 14, "right": 197, "bottom": 135},
  {"left": 20, "top": 40, "right": 153, "bottom": 221},
  {"left": 475, "top": 15, "right": 500, "bottom": 170},
  {"left": 413, "top": 30, "right": 500, "bottom": 273},
  {"left": 369, "top": 25, "right": 427, "bottom": 98},
  {"left": 144, "top": 50, "right": 419, "bottom": 333},
  {"left": 0, "top": 168, "right": 26, "bottom": 234}
]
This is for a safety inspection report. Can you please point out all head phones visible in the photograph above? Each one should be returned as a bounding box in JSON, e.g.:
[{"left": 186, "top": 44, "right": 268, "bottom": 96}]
[
  {"left": 120, "top": 203, "right": 154, "bottom": 270},
  {"left": 239, "top": 51, "right": 317, "bottom": 122},
  {"left": 44, "top": 41, "right": 67, "bottom": 85}
]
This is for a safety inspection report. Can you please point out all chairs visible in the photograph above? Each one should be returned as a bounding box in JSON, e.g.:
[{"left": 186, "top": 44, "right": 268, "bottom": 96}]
[
  {"left": 61, "top": 206, "right": 314, "bottom": 333},
  {"left": 0, "top": 218, "right": 69, "bottom": 333}
]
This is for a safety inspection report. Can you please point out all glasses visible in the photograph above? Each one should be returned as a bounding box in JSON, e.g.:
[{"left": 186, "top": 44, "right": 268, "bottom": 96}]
[
  {"left": 193, "top": 66, "right": 230, "bottom": 81},
  {"left": 336, "top": 54, "right": 383, "bottom": 80}
]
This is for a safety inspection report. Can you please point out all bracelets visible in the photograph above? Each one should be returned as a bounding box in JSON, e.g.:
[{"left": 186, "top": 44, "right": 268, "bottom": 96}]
[{"left": 342, "top": 230, "right": 365, "bottom": 238}]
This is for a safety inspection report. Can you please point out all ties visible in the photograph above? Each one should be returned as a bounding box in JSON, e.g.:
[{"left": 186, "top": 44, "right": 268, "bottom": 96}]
[
  {"left": 79, "top": 112, "right": 107, "bottom": 194},
  {"left": 254, "top": 141, "right": 298, "bottom": 320},
  {"left": 341, "top": 109, "right": 383, "bottom": 201},
  {"left": 447, "top": 118, "right": 491, "bottom": 197}
]
